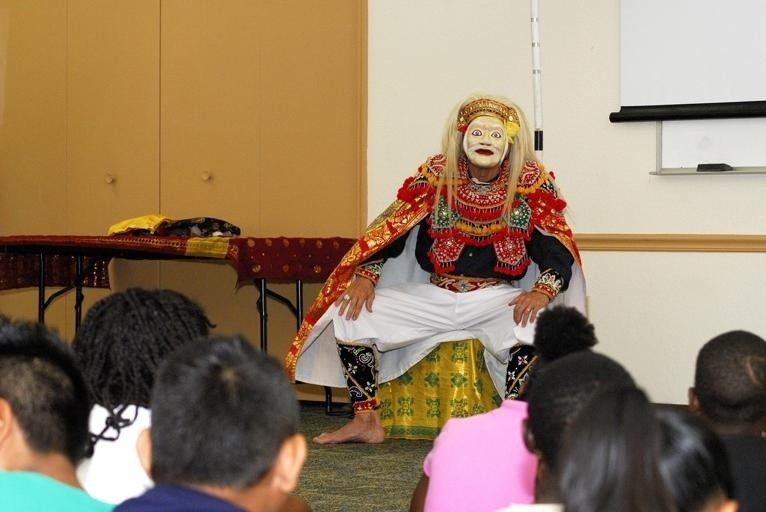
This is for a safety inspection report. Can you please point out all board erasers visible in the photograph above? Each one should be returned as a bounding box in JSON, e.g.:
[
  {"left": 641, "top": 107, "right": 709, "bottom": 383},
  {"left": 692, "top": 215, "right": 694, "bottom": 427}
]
[{"left": 696, "top": 164, "right": 732, "bottom": 171}]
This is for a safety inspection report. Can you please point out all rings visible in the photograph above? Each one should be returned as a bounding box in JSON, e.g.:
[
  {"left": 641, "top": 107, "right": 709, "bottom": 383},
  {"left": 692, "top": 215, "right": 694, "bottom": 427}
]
[
  {"left": 527, "top": 308, "right": 530, "bottom": 312},
  {"left": 343, "top": 294, "right": 350, "bottom": 301}
]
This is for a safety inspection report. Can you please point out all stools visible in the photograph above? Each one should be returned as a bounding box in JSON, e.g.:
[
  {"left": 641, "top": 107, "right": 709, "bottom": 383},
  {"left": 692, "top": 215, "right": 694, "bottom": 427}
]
[{"left": 376, "top": 339, "right": 502, "bottom": 440}]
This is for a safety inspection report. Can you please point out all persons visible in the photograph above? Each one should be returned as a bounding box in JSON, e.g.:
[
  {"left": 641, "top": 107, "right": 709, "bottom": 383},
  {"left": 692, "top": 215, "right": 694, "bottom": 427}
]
[
  {"left": 560, "top": 386, "right": 740, "bottom": 512},
  {"left": 686, "top": 329, "right": 766, "bottom": 512},
  {"left": 0, "top": 309, "right": 114, "bottom": 512},
  {"left": 284, "top": 93, "right": 590, "bottom": 446},
  {"left": 114, "top": 334, "right": 308, "bottom": 511},
  {"left": 69, "top": 286, "right": 219, "bottom": 505},
  {"left": 499, "top": 351, "right": 641, "bottom": 512},
  {"left": 410, "top": 303, "right": 599, "bottom": 512}
]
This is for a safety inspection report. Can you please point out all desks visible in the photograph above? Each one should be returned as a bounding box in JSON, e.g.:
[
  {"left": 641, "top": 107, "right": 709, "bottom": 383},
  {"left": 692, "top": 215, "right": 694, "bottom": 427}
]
[{"left": 1, "top": 236, "right": 358, "bottom": 415}]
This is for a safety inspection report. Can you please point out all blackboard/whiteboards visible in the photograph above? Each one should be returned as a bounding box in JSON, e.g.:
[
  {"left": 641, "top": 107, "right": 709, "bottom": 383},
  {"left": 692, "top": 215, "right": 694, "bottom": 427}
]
[{"left": 656, "top": 117, "right": 766, "bottom": 175}]
